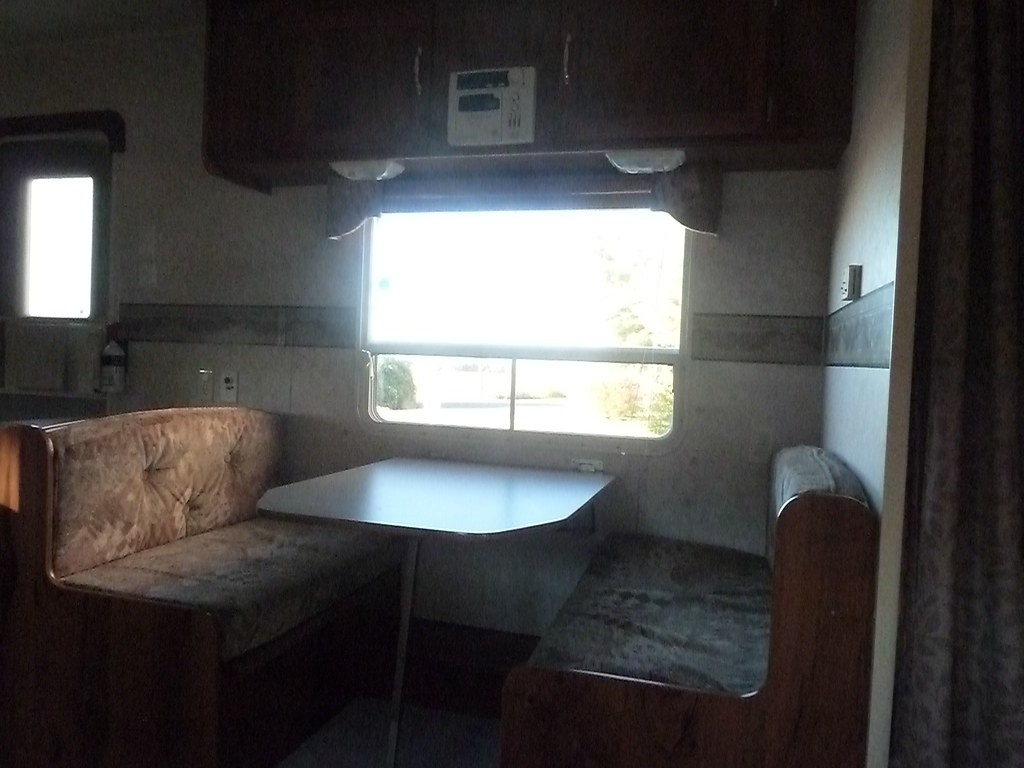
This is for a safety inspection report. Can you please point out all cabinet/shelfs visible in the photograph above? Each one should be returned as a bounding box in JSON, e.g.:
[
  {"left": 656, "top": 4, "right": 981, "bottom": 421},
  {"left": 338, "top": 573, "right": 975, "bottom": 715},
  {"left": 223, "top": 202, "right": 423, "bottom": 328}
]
[
  {"left": 203, "top": 1, "right": 427, "bottom": 199},
  {"left": 434, "top": 0, "right": 863, "bottom": 177}
]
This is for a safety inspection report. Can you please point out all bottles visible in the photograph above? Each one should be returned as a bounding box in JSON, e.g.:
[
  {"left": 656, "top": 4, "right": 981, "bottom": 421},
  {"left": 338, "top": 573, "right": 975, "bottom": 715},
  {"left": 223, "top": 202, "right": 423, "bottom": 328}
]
[{"left": 100, "top": 341, "right": 126, "bottom": 393}]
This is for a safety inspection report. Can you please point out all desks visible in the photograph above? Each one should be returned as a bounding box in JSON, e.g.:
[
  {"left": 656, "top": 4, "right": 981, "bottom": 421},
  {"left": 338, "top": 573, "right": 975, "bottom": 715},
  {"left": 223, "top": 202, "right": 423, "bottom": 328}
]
[{"left": 255, "top": 452, "right": 618, "bottom": 765}]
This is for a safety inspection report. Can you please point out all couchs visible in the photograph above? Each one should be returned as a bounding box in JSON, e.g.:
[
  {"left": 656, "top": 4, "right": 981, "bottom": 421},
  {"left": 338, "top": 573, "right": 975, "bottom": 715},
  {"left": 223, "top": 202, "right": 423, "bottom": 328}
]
[
  {"left": 497, "top": 443, "right": 883, "bottom": 763},
  {"left": 3, "top": 405, "right": 411, "bottom": 768}
]
